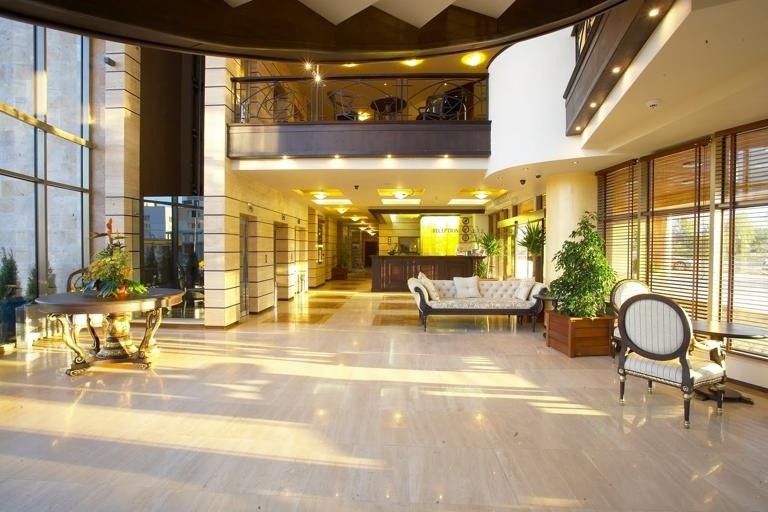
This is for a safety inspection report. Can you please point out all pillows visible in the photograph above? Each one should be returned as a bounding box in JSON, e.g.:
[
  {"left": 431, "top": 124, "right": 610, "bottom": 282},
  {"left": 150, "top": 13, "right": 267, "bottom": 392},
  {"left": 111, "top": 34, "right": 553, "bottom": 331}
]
[
  {"left": 513, "top": 277, "right": 535, "bottom": 301},
  {"left": 452, "top": 276, "right": 481, "bottom": 298},
  {"left": 418, "top": 272, "right": 440, "bottom": 300}
]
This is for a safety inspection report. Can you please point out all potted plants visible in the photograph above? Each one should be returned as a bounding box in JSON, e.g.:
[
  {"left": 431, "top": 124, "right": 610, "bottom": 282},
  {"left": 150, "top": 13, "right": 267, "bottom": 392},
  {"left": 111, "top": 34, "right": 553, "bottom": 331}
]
[{"left": 547, "top": 210, "right": 620, "bottom": 358}]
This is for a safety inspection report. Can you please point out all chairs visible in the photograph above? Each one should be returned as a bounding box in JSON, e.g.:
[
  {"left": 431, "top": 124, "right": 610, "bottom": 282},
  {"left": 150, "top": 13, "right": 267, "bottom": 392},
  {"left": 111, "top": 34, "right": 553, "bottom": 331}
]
[
  {"left": 327, "top": 88, "right": 358, "bottom": 120},
  {"left": 415, "top": 89, "right": 466, "bottom": 121},
  {"left": 617, "top": 292, "right": 727, "bottom": 428},
  {"left": 611, "top": 277, "right": 657, "bottom": 396}
]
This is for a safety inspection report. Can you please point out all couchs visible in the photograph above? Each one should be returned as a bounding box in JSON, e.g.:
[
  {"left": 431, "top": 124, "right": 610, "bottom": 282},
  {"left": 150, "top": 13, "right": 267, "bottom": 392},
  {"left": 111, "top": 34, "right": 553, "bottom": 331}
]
[{"left": 407, "top": 276, "right": 549, "bottom": 331}]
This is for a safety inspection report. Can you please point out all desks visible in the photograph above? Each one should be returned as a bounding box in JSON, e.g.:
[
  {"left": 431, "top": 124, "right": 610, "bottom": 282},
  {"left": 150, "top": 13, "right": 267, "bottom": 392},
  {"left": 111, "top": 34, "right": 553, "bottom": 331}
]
[
  {"left": 691, "top": 318, "right": 768, "bottom": 404},
  {"left": 533, "top": 292, "right": 567, "bottom": 338},
  {"left": 370, "top": 95, "right": 407, "bottom": 121},
  {"left": 35, "top": 288, "right": 185, "bottom": 377}
]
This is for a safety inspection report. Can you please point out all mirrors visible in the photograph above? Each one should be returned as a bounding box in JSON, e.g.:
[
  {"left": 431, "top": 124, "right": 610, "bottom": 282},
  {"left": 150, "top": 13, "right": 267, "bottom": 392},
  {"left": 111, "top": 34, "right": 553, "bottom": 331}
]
[{"left": 398, "top": 236, "right": 420, "bottom": 254}]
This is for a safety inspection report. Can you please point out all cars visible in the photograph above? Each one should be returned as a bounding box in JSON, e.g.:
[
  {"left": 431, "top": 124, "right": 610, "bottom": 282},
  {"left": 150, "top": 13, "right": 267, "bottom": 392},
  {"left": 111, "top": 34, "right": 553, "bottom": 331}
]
[{"left": 671, "top": 254, "right": 693, "bottom": 271}]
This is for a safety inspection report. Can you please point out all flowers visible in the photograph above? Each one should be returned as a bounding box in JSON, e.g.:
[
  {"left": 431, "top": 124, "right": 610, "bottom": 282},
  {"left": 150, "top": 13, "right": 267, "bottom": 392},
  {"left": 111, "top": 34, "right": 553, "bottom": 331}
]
[{"left": 78, "top": 219, "right": 148, "bottom": 296}]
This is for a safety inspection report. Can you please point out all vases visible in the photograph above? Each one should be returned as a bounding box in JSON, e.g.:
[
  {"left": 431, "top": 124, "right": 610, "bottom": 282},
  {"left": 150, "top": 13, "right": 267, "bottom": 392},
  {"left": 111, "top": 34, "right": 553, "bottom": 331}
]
[{"left": 109, "top": 280, "right": 127, "bottom": 295}]
